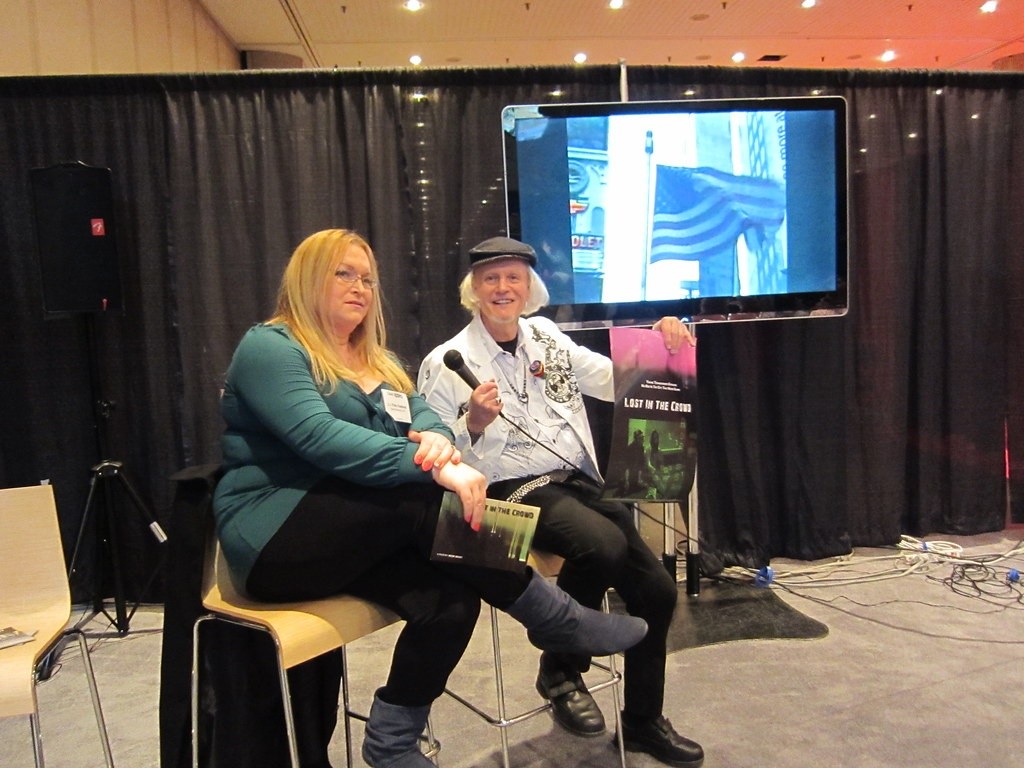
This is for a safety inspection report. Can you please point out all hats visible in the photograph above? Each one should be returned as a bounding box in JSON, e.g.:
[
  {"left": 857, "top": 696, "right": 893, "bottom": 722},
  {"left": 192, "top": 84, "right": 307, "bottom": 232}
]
[{"left": 469, "top": 235, "right": 535, "bottom": 268}]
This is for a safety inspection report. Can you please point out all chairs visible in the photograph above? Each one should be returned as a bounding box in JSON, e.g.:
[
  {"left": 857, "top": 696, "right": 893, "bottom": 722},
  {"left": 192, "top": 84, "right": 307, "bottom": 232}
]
[
  {"left": 174, "top": 467, "right": 442, "bottom": 768},
  {"left": 0, "top": 481, "right": 112, "bottom": 768},
  {"left": 442, "top": 541, "right": 626, "bottom": 768}
]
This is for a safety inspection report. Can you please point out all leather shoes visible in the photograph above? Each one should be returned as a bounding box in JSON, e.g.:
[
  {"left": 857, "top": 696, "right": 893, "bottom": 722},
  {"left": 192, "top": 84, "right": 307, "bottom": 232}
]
[
  {"left": 535, "top": 665, "right": 607, "bottom": 736},
  {"left": 614, "top": 710, "right": 704, "bottom": 768}
]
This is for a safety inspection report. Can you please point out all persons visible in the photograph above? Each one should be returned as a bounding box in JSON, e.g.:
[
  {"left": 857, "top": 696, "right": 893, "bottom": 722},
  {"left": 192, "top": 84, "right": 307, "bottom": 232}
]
[
  {"left": 614, "top": 428, "right": 664, "bottom": 493},
  {"left": 211, "top": 228, "right": 648, "bottom": 768},
  {"left": 417, "top": 236, "right": 705, "bottom": 768}
]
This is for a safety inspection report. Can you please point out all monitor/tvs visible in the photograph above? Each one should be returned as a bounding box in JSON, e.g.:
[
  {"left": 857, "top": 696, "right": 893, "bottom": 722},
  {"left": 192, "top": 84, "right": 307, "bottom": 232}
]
[{"left": 500, "top": 94, "right": 851, "bottom": 329}]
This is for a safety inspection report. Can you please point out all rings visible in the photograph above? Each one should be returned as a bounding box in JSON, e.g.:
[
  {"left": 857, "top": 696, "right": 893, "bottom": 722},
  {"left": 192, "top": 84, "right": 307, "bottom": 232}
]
[
  {"left": 451, "top": 445, "right": 456, "bottom": 451},
  {"left": 496, "top": 398, "right": 501, "bottom": 404}
]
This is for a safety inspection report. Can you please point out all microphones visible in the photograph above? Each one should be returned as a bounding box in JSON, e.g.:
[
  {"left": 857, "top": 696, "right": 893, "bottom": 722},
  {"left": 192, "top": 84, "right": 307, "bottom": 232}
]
[{"left": 443, "top": 349, "right": 481, "bottom": 392}]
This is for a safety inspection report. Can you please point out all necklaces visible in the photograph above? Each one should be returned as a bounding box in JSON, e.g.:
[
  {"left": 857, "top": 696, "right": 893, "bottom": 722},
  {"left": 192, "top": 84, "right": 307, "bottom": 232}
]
[{"left": 508, "top": 354, "right": 529, "bottom": 403}]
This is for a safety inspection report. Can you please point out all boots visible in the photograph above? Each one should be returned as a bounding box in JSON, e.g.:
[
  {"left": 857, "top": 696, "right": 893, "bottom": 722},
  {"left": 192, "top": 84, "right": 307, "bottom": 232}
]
[
  {"left": 501, "top": 564, "right": 649, "bottom": 657},
  {"left": 361, "top": 686, "right": 439, "bottom": 768}
]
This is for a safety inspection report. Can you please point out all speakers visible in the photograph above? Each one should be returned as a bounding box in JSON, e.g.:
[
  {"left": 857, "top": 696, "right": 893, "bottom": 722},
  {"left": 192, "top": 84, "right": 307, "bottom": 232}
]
[{"left": 22, "top": 160, "right": 126, "bottom": 317}]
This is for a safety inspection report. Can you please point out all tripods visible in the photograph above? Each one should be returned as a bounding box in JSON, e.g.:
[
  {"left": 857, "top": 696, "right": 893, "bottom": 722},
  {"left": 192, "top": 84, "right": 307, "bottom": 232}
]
[{"left": 52, "top": 310, "right": 174, "bottom": 638}]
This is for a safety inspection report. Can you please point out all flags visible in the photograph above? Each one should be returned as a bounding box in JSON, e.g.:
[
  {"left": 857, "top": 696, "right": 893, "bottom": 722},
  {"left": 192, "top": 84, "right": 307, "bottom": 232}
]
[{"left": 650, "top": 165, "right": 785, "bottom": 263}]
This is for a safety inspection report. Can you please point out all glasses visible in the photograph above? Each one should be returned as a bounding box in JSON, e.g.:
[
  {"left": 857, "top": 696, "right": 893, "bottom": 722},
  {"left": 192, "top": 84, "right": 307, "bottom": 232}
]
[{"left": 335, "top": 271, "right": 379, "bottom": 290}]
